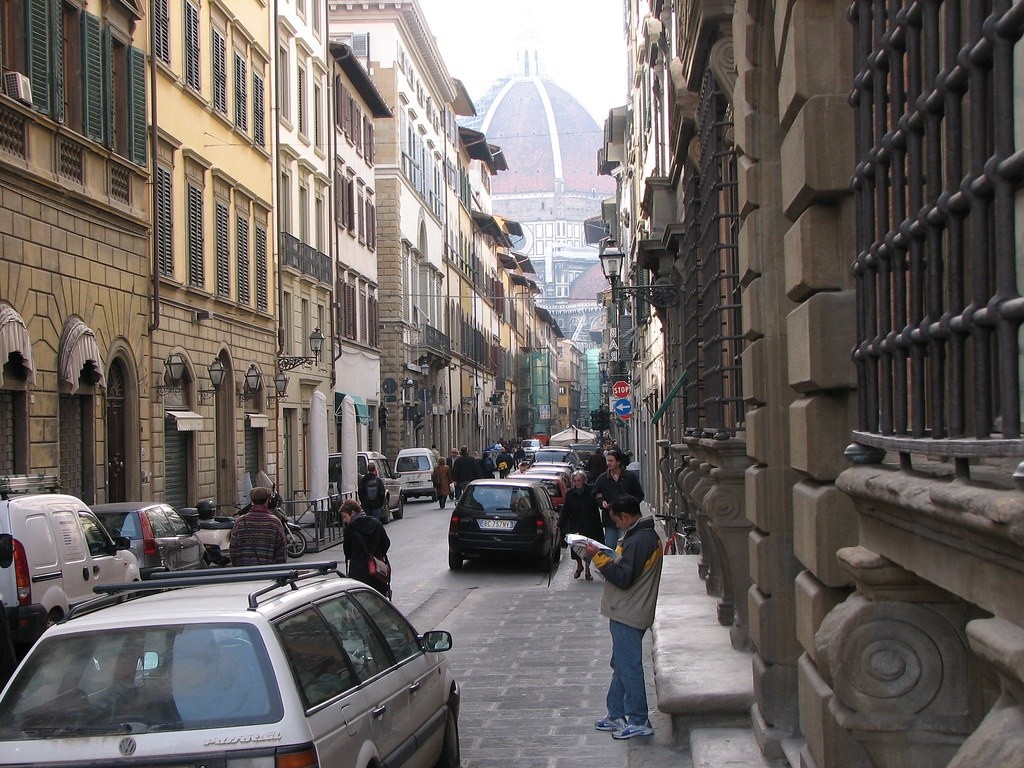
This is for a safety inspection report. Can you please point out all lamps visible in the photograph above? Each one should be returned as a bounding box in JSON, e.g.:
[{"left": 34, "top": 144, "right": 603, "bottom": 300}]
[
  {"left": 277, "top": 325, "right": 326, "bottom": 373},
  {"left": 598, "top": 238, "right": 673, "bottom": 315},
  {"left": 198, "top": 357, "right": 228, "bottom": 402},
  {"left": 266, "top": 370, "right": 291, "bottom": 404},
  {"left": 239, "top": 363, "right": 263, "bottom": 403},
  {"left": 151, "top": 353, "right": 186, "bottom": 397}
]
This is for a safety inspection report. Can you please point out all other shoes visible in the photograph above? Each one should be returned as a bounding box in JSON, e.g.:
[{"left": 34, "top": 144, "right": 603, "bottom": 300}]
[
  {"left": 574, "top": 566, "right": 584, "bottom": 578},
  {"left": 585, "top": 574, "right": 593, "bottom": 580}
]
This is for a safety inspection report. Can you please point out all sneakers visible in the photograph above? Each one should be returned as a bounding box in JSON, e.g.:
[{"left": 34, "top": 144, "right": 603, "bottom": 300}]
[
  {"left": 611, "top": 718, "right": 654, "bottom": 739},
  {"left": 594, "top": 715, "right": 628, "bottom": 730}
]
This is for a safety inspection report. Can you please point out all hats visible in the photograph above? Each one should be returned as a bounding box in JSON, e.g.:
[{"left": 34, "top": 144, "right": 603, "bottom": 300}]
[{"left": 251, "top": 487, "right": 271, "bottom": 500}]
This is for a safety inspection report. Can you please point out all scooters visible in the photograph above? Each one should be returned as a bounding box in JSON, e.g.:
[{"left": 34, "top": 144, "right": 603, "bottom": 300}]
[{"left": 180, "top": 469, "right": 307, "bottom": 567}]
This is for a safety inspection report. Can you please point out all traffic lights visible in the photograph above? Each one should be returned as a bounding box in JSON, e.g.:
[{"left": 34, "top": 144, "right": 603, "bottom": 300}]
[{"left": 590, "top": 410, "right": 604, "bottom": 431}]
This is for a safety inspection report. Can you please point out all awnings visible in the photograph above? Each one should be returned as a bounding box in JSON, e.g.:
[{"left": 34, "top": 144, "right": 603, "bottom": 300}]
[
  {"left": 246, "top": 413, "right": 269, "bottom": 428},
  {"left": 335, "top": 391, "right": 369, "bottom": 425},
  {"left": 168, "top": 411, "right": 204, "bottom": 431}
]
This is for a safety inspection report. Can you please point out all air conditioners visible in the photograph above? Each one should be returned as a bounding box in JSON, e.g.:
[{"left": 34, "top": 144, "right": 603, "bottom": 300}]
[{"left": 4, "top": 72, "right": 34, "bottom": 107}]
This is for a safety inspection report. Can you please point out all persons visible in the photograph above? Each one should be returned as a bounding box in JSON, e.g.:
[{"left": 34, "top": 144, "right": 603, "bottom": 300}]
[
  {"left": 585, "top": 493, "right": 663, "bottom": 738},
  {"left": 445, "top": 448, "right": 461, "bottom": 501},
  {"left": 228, "top": 486, "right": 288, "bottom": 566},
  {"left": 496, "top": 447, "right": 513, "bottom": 480},
  {"left": 586, "top": 432, "right": 640, "bottom": 484},
  {"left": 433, "top": 457, "right": 451, "bottom": 508},
  {"left": 499, "top": 437, "right": 525, "bottom": 471},
  {"left": 359, "top": 462, "right": 385, "bottom": 520},
  {"left": 592, "top": 450, "right": 645, "bottom": 552},
  {"left": 431, "top": 445, "right": 440, "bottom": 459},
  {"left": 453, "top": 445, "right": 496, "bottom": 505},
  {"left": 340, "top": 500, "right": 392, "bottom": 598},
  {"left": 558, "top": 471, "right": 604, "bottom": 581}
]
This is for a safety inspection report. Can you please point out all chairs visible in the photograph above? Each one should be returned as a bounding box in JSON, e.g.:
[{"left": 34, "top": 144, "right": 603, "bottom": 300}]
[
  {"left": 161, "top": 630, "right": 222, "bottom": 688},
  {"left": 480, "top": 492, "right": 495, "bottom": 504}
]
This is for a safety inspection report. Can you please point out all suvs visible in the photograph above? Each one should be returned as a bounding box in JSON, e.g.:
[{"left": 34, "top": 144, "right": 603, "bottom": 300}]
[
  {"left": 0, "top": 473, "right": 144, "bottom": 644},
  {"left": 0, "top": 559, "right": 462, "bottom": 768},
  {"left": 328, "top": 451, "right": 404, "bottom": 525}
]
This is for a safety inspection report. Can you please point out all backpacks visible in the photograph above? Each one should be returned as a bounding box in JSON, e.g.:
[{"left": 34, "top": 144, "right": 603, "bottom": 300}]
[{"left": 363, "top": 476, "right": 380, "bottom": 502}]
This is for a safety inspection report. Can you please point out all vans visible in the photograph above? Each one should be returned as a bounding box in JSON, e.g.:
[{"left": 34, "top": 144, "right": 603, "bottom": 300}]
[{"left": 393, "top": 447, "right": 439, "bottom": 501}]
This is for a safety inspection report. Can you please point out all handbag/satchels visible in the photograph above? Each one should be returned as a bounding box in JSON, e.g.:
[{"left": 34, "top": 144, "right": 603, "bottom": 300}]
[
  {"left": 432, "top": 473, "right": 440, "bottom": 488},
  {"left": 368, "top": 556, "right": 390, "bottom": 584},
  {"left": 497, "top": 462, "right": 508, "bottom": 471},
  {"left": 449, "top": 482, "right": 456, "bottom": 493}
]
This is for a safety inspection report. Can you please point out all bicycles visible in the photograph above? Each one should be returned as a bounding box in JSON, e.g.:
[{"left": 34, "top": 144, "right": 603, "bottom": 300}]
[{"left": 655, "top": 513, "right": 698, "bottom": 555}]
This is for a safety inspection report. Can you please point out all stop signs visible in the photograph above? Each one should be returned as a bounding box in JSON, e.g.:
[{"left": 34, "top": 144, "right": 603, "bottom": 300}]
[{"left": 612, "top": 381, "right": 631, "bottom": 398}]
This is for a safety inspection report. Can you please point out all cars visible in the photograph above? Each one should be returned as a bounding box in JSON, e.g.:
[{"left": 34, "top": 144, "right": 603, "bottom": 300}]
[
  {"left": 520, "top": 423, "right": 551, "bottom": 457},
  {"left": 448, "top": 444, "right": 581, "bottom": 570},
  {"left": 88, "top": 501, "right": 210, "bottom": 578}
]
